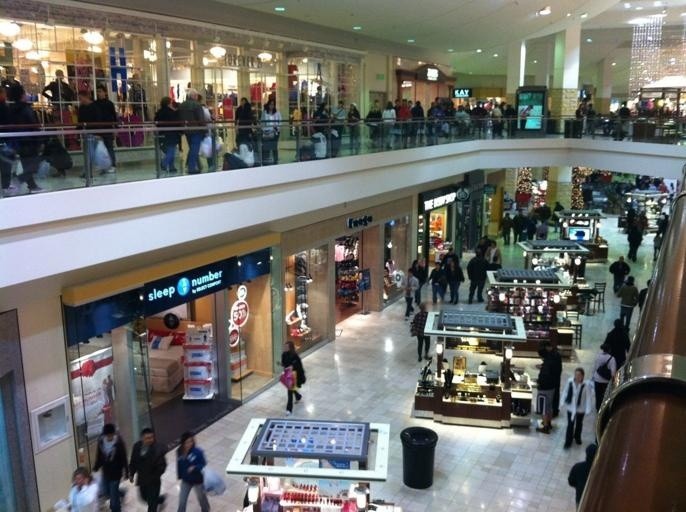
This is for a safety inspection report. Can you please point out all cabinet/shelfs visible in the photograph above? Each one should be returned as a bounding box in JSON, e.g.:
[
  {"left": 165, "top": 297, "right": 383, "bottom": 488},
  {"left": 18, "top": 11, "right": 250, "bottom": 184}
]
[
  {"left": 623, "top": 189, "right": 668, "bottom": 233},
  {"left": 411, "top": 311, "right": 532, "bottom": 428},
  {"left": 479, "top": 208, "right": 610, "bottom": 352}
]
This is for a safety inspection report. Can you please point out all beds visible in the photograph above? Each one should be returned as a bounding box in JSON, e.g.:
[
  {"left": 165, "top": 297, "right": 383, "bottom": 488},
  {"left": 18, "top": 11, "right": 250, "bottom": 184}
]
[{"left": 132, "top": 316, "right": 206, "bottom": 393}]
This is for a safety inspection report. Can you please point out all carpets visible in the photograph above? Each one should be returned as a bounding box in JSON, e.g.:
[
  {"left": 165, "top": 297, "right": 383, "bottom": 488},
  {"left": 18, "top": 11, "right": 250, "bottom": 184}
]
[{"left": 134, "top": 389, "right": 241, "bottom": 451}]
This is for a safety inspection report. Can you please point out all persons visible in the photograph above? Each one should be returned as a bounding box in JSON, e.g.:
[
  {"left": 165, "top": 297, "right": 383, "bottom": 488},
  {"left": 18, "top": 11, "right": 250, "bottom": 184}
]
[
  {"left": 177, "top": 432, "right": 211, "bottom": 512},
  {"left": 276, "top": 341, "right": 306, "bottom": 417},
  {"left": 58, "top": 467, "right": 99, "bottom": 512},
  {"left": 93, "top": 424, "right": 129, "bottom": 512},
  {"left": 128, "top": 427, "right": 168, "bottom": 512}
]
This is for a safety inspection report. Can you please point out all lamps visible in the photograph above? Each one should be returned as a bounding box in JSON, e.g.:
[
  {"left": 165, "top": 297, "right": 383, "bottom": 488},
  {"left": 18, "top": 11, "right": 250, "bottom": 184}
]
[
  {"left": 245, "top": 478, "right": 260, "bottom": 506},
  {"left": 353, "top": 486, "right": 369, "bottom": 511}
]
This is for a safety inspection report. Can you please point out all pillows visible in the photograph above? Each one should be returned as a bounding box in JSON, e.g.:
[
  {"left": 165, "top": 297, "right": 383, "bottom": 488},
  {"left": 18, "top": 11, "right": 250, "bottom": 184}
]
[{"left": 148, "top": 334, "right": 174, "bottom": 352}]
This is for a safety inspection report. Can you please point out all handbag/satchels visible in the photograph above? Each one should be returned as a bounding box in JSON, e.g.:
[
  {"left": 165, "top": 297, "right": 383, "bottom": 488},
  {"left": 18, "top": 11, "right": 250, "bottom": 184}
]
[
  {"left": 279, "top": 367, "right": 294, "bottom": 390},
  {"left": 261, "top": 127, "right": 277, "bottom": 140},
  {"left": 596, "top": 361, "right": 615, "bottom": 381}
]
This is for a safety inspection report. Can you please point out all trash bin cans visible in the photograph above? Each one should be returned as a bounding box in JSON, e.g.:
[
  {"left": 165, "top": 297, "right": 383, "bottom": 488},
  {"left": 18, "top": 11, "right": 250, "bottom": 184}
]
[{"left": 400, "top": 427, "right": 439, "bottom": 489}]
